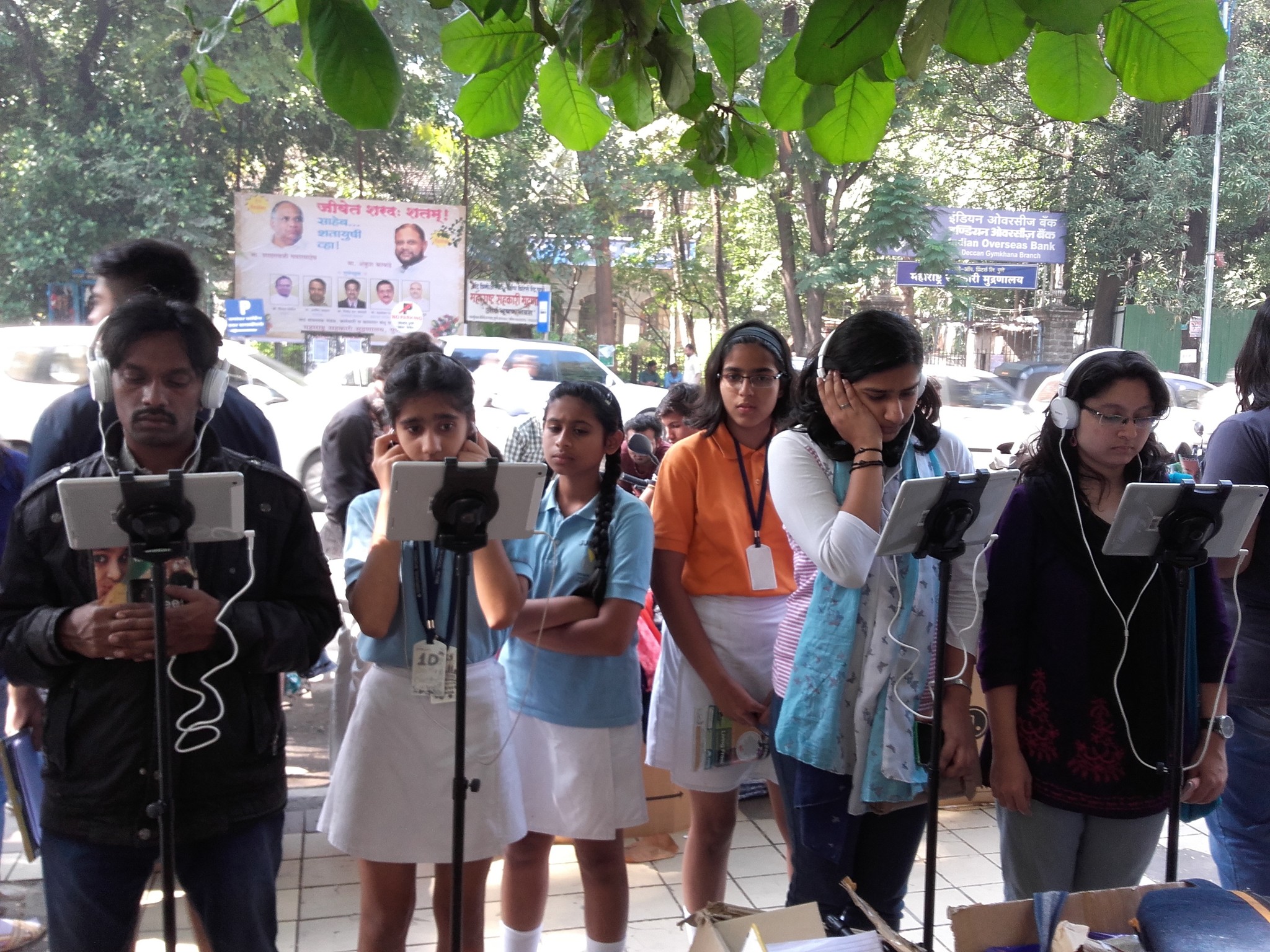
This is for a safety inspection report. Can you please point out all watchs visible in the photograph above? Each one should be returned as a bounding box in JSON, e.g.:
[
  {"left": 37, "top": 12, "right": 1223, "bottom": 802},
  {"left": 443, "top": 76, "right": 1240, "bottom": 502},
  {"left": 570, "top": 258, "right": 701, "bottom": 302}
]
[{"left": 1201, "top": 716, "right": 1235, "bottom": 739}]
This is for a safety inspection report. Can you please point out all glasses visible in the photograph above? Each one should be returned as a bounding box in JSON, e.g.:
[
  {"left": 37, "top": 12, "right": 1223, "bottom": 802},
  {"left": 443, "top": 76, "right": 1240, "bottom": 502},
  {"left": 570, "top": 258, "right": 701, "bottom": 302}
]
[
  {"left": 1078, "top": 404, "right": 1162, "bottom": 431},
  {"left": 715, "top": 368, "right": 788, "bottom": 387}
]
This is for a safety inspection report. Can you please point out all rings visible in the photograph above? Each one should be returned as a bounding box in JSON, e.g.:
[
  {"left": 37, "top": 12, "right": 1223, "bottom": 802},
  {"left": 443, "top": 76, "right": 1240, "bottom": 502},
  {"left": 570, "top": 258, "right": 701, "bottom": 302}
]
[{"left": 840, "top": 403, "right": 850, "bottom": 410}]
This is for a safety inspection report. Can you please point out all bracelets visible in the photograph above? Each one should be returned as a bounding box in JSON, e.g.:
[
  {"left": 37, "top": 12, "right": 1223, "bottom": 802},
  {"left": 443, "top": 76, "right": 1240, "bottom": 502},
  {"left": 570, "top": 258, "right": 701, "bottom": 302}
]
[
  {"left": 849, "top": 448, "right": 885, "bottom": 474},
  {"left": 945, "top": 678, "right": 972, "bottom": 694}
]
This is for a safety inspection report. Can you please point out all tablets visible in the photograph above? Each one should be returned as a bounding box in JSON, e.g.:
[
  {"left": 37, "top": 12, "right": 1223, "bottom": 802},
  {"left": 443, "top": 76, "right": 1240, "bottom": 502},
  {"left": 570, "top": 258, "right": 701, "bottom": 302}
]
[
  {"left": 386, "top": 460, "right": 548, "bottom": 541},
  {"left": 56, "top": 469, "right": 244, "bottom": 550},
  {"left": 1102, "top": 481, "right": 1269, "bottom": 559},
  {"left": 873, "top": 469, "right": 1021, "bottom": 557}
]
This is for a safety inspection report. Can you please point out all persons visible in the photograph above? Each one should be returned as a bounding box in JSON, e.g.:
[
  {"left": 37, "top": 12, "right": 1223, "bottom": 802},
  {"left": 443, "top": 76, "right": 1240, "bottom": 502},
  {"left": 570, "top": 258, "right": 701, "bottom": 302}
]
[
  {"left": 267, "top": 201, "right": 427, "bottom": 307},
  {"left": 645, "top": 318, "right": 797, "bottom": 920},
  {"left": 0, "top": 238, "right": 342, "bottom": 951},
  {"left": 315, "top": 353, "right": 536, "bottom": 951},
  {"left": 981, "top": 351, "right": 1233, "bottom": 902},
  {"left": 499, "top": 378, "right": 653, "bottom": 952},
  {"left": 768, "top": 310, "right": 981, "bottom": 931},
  {"left": 324, "top": 330, "right": 974, "bottom": 524},
  {"left": 1199, "top": 301, "right": 1269, "bottom": 898}
]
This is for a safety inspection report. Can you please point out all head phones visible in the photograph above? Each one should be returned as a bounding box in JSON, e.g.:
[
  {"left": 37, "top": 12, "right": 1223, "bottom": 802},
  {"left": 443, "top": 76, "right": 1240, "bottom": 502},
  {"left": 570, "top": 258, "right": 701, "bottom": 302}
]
[
  {"left": 820, "top": 327, "right": 927, "bottom": 403},
  {"left": 1050, "top": 347, "right": 1131, "bottom": 430},
  {"left": 85, "top": 298, "right": 231, "bottom": 409}
]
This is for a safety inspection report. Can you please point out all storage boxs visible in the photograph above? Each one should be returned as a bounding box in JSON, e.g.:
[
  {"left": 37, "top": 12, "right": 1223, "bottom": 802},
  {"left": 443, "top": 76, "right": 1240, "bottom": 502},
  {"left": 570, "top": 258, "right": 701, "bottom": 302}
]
[
  {"left": 681, "top": 874, "right": 931, "bottom": 952},
  {"left": 949, "top": 878, "right": 1267, "bottom": 951},
  {"left": 547, "top": 738, "right": 689, "bottom": 844}
]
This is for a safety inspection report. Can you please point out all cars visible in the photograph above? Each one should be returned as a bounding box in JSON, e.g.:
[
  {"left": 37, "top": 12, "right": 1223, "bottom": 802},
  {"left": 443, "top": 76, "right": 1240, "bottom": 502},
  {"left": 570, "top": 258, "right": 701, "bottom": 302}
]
[{"left": 1, "top": 327, "right": 1269, "bottom": 511}]
